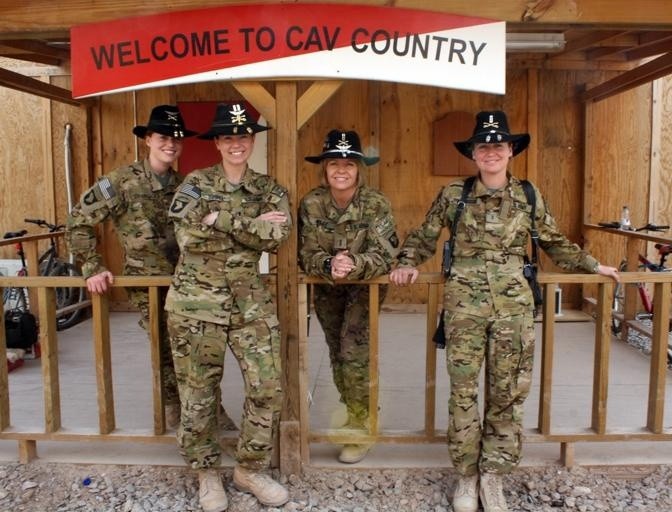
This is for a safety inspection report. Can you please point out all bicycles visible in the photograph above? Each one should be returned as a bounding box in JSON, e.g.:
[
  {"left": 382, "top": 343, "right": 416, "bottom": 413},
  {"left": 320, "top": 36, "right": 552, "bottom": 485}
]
[
  {"left": 0, "top": 218, "right": 85, "bottom": 331},
  {"left": 598, "top": 219, "right": 672, "bottom": 369}
]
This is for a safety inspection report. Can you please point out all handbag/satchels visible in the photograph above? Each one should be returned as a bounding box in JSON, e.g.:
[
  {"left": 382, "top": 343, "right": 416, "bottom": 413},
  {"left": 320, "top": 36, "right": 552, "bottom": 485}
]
[{"left": 5, "top": 309, "right": 37, "bottom": 350}]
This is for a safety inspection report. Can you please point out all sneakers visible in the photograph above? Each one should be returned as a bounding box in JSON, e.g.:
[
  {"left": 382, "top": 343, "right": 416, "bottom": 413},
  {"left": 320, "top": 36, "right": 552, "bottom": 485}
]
[
  {"left": 480, "top": 474, "right": 508, "bottom": 512},
  {"left": 216, "top": 412, "right": 240, "bottom": 457},
  {"left": 452, "top": 476, "right": 479, "bottom": 512},
  {"left": 166, "top": 405, "right": 182, "bottom": 428},
  {"left": 233, "top": 464, "right": 288, "bottom": 506},
  {"left": 198, "top": 469, "right": 228, "bottom": 512}
]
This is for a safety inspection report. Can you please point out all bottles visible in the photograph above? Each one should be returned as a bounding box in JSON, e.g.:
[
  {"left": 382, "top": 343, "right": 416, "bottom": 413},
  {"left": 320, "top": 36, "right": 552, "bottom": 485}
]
[{"left": 620, "top": 206, "right": 631, "bottom": 231}]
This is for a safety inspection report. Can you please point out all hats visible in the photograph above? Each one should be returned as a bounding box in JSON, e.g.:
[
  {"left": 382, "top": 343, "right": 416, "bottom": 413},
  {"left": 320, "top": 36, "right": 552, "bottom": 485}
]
[
  {"left": 199, "top": 102, "right": 271, "bottom": 140},
  {"left": 453, "top": 110, "right": 530, "bottom": 156},
  {"left": 133, "top": 105, "right": 197, "bottom": 139},
  {"left": 304, "top": 130, "right": 379, "bottom": 166}
]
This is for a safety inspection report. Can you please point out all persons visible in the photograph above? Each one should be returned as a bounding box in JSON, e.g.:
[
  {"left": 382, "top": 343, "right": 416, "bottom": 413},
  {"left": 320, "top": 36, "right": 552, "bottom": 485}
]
[
  {"left": 162, "top": 98, "right": 294, "bottom": 512},
  {"left": 62, "top": 103, "right": 241, "bottom": 433},
  {"left": 297, "top": 128, "right": 400, "bottom": 465},
  {"left": 387, "top": 109, "right": 620, "bottom": 511}
]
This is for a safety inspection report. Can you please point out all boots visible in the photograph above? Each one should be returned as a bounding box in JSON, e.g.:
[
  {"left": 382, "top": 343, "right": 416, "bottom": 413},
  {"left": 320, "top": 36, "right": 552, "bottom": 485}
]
[{"left": 339, "top": 406, "right": 381, "bottom": 463}]
